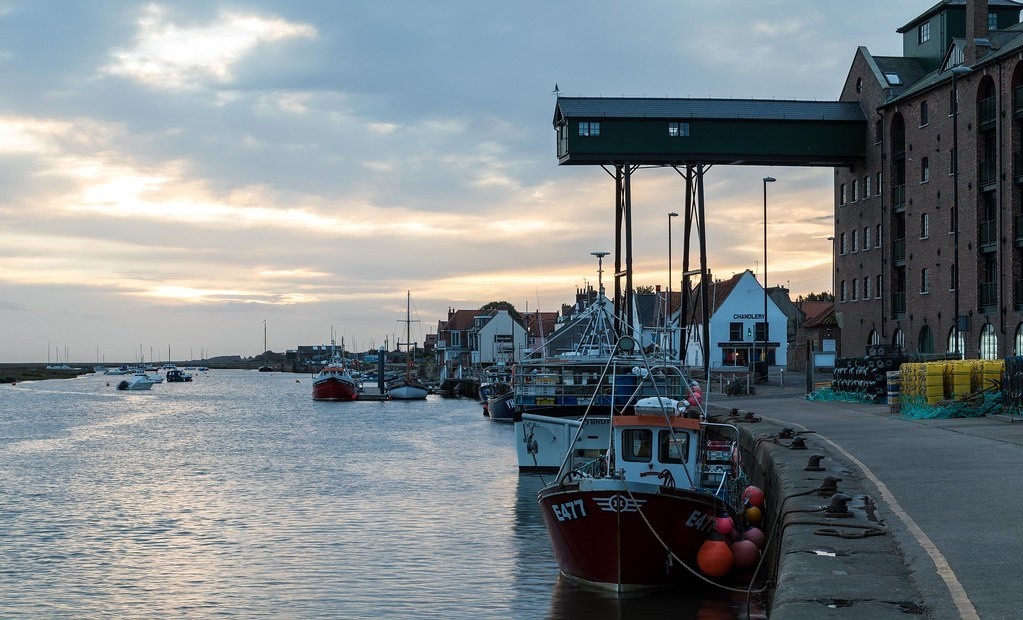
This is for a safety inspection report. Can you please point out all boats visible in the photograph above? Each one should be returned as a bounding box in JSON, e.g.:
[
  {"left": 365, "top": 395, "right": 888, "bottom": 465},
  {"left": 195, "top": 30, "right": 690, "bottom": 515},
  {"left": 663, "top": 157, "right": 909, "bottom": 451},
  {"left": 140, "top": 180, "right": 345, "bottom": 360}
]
[
  {"left": 115, "top": 377, "right": 155, "bottom": 391},
  {"left": 486, "top": 294, "right": 766, "bottom": 595}
]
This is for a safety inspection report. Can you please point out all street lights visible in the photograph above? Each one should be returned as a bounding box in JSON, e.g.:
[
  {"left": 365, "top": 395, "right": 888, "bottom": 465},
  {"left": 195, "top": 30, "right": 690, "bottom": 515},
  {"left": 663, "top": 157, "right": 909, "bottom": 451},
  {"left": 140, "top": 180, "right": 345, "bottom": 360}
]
[
  {"left": 667, "top": 212, "right": 680, "bottom": 354},
  {"left": 951, "top": 65, "right": 974, "bottom": 352},
  {"left": 591, "top": 250, "right": 610, "bottom": 296},
  {"left": 763, "top": 175, "right": 777, "bottom": 341}
]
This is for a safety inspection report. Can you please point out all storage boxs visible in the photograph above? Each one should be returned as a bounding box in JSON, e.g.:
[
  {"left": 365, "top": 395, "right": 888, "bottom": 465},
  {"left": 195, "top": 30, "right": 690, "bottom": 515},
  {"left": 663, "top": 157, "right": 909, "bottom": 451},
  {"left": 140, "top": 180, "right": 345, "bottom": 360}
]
[
  {"left": 707, "top": 441, "right": 733, "bottom": 480},
  {"left": 514, "top": 372, "right": 636, "bottom": 406},
  {"left": 831, "top": 343, "right": 1005, "bottom": 407}
]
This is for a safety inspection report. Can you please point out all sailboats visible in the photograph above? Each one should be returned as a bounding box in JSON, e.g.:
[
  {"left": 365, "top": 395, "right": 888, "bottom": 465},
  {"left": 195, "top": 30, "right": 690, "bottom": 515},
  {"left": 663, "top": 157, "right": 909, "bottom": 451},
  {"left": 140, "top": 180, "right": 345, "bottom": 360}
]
[
  {"left": 45, "top": 343, "right": 210, "bottom": 383},
  {"left": 312, "top": 288, "right": 428, "bottom": 400},
  {"left": 259, "top": 319, "right": 274, "bottom": 371}
]
